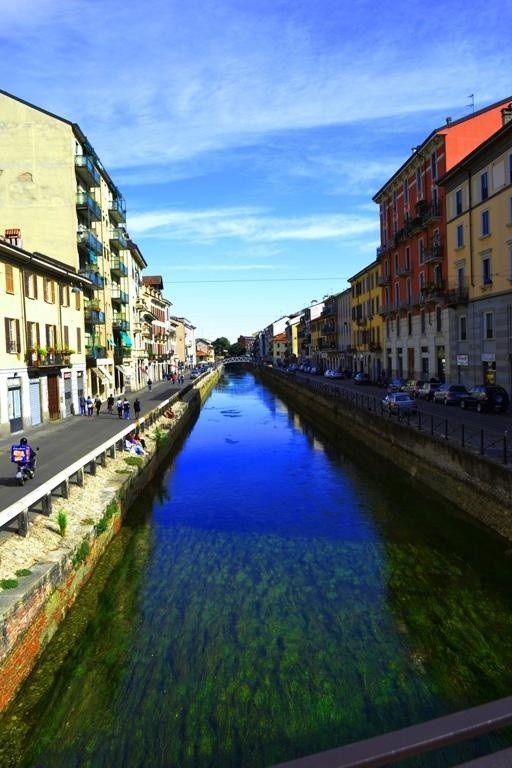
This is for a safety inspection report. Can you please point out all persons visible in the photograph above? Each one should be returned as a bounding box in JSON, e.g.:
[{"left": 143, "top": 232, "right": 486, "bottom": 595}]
[
  {"left": 163, "top": 371, "right": 185, "bottom": 385},
  {"left": 76, "top": 394, "right": 141, "bottom": 420},
  {"left": 147, "top": 378, "right": 152, "bottom": 392},
  {"left": 20, "top": 438, "right": 35, "bottom": 467}
]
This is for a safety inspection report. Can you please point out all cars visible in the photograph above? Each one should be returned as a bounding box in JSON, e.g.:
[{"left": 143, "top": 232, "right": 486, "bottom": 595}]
[
  {"left": 287, "top": 362, "right": 321, "bottom": 376},
  {"left": 354, "top": 373, "right": 371, "bottom": 386},
  {"left": 381, "top": 393, "right": 417, "bottom": 415},
  {"left": 386, "top": 377, "right": 467, "bottom": 404}
]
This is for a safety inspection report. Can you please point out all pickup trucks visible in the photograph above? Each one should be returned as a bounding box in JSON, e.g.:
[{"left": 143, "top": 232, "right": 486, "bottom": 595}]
[{"left": 324, "top": 369, "right": 345, "bottom": 380}]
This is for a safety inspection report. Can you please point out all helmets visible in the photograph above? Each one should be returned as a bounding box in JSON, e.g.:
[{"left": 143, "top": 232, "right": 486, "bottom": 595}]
[{"left": 20, "top": 438, "right": 27, "bottom": 444}]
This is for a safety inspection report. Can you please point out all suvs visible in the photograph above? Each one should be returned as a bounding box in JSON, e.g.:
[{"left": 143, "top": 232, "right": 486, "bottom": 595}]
[{"left": 461, "top": 384, "right": 509, "bottom": 414}]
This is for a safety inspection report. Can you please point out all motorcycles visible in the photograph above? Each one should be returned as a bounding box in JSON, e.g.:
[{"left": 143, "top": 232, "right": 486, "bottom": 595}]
[{"left": 14, "top": 447, "right": 39, "bottom": 485}]
